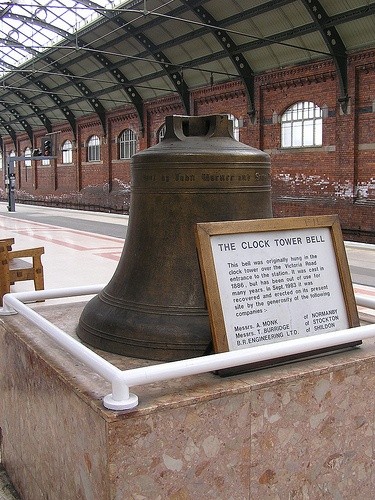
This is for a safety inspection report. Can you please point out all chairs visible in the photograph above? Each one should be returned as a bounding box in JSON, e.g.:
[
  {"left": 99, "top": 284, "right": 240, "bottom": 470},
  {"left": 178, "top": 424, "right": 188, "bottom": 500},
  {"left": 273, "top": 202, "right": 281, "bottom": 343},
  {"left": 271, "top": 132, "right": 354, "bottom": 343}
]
[{"left": 0, "top": 238, "right": 45, "bottom": 307}]
